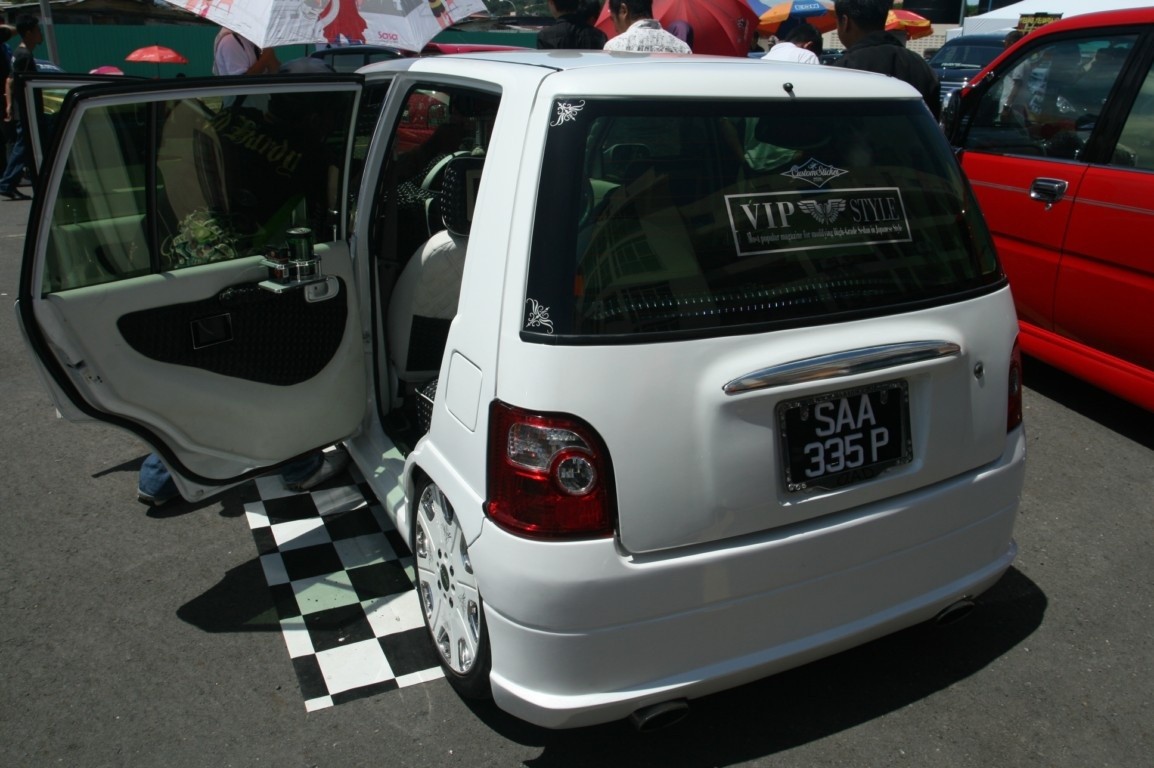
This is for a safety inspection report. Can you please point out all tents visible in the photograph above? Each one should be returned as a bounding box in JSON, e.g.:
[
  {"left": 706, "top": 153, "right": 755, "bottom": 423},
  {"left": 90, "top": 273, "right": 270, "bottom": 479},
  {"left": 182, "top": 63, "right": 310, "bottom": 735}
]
[{"left": 964, "top": 0, "right": 1154, "bottom": 37}]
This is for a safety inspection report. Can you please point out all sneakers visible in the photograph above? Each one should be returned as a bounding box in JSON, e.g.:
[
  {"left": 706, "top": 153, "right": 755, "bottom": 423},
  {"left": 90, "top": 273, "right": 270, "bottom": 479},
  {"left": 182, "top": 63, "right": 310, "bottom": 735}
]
[
  {"left": 137, "top": 488, "right": 183, "bottom": 506},
  {"left": 283, "top": 447, "right": 351, "bottom": 491},
  {"left": 3, "top": 189, "right": 31, "bottom": 201}
]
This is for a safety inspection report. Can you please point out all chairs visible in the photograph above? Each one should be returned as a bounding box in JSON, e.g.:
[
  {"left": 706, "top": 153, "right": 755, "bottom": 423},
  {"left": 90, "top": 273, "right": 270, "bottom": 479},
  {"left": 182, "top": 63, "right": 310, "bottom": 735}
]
[
  {"left": 387, "top": 155, "right": 488, "bottom": 396},
  {"left": 978, "top": 48, "right": 1002, "bottom": 65}
]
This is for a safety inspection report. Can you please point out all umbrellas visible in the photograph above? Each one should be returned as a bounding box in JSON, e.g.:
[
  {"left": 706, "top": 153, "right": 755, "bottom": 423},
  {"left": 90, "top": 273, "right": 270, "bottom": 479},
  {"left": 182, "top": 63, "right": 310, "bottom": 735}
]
[
  {"left": 884, "top": 10, "right": 933, "bottom": 40},
  {"left": 755, "top": 0, "right": 838, "bottom": 37},
  {"left": 163, "top": 0, "right": 488, "bottom": 53},
  {"left": 125, "top": 46, "right": 189, "bottom": 77},
  {"left": 89, "top": 65, "right": 125, "bottom": 75},
  {"left": 593, "top": 0, "right": 762, "bottom": 56},
  {"left": 11, "top": 56, "right": 66, "bottom": 72}
]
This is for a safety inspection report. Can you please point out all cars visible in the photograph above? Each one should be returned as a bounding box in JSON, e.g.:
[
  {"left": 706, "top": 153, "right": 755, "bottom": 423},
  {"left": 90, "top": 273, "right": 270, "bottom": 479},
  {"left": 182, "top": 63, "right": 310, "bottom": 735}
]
[
  {"left": 927, "top": 33, "right": 1013, "bottom": 125},
  {"left": 13, "top": 0, "right": 1030, "bottom": 732},
  {"left": 932, "top": 5, "right": 1154, "bottom": 409}
]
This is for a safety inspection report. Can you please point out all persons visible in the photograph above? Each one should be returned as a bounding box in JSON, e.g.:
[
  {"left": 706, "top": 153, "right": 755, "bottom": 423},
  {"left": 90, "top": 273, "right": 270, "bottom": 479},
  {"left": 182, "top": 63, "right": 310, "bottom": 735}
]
[{"left": 0, "top": 0, "right": 1132, "bottom": 509}]
